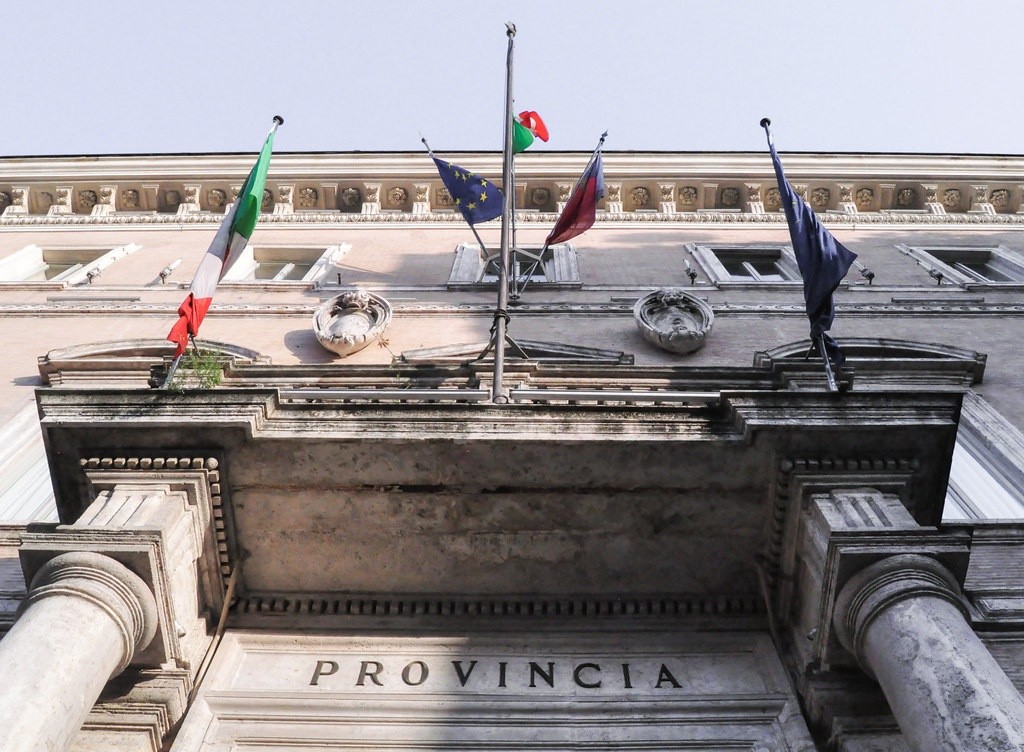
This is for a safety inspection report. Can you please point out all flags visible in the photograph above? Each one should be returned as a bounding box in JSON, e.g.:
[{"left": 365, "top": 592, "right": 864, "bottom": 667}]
[
  {"left": 769, "top": 147, "right": 856, "bottom": 365},
  {"left": 431, "top": 155, "right": 505, "bottom": 224},
  {"left": 166, "top": 132, "right": 272, "bottom": 360},
  {"left": 546, "top": 151, "right": 606, "bottom": 245},
  {"left": 513, "top": 110, "right": 549, "bottom": 157}
]
